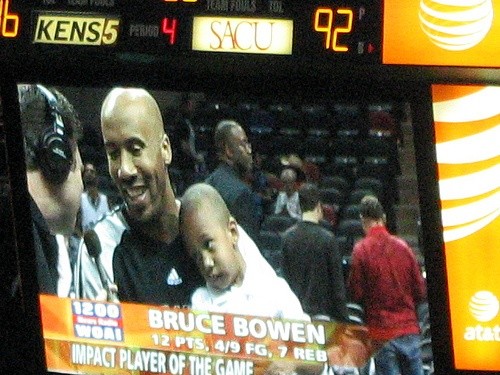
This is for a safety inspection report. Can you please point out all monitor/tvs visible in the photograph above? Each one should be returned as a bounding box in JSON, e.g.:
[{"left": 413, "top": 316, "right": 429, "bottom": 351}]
[{"left": 2, "top": 68, "right": 451, "bottom": 375}]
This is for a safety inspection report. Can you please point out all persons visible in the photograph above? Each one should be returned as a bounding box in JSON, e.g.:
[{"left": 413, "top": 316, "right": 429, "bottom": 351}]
[
  {"left": 280, "top": 184, "right": 349, "bottom": 348},
  {"left": 17, "top": 82, "right": 86, "bottom": 300},
  {"left": 169, "top": 86, "right": 317, "bottom": 252},
  {"left": 179, "top": 183, "right": 335, "bottom": 375},
  {"left": 75, "top": 163, "right": 113, "bottom": 239},
  {"left": 349, "top": 195, "right": 430, "bottom": 375},
  {"left": 73, "top": 86, "right": 277, "bottom": 311}
]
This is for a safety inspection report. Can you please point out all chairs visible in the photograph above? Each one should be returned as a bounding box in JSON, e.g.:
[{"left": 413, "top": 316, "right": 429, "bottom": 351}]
[{"left": 165, "top": 98, "right": 408, "bottom": 374}]
